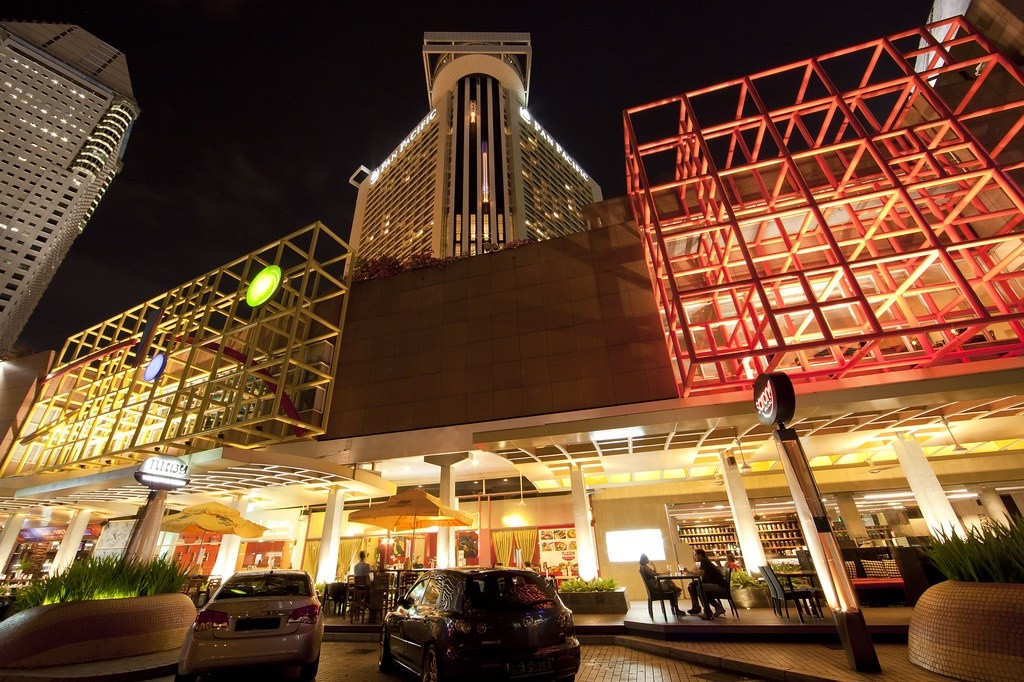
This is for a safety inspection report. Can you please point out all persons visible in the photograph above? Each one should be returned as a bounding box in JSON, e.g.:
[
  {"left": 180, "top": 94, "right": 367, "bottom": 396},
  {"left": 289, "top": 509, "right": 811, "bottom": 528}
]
[
  {"left": 687, "top": 549, "right": 728, "bottom": 620},
  {"left": 639, "top": 554, "right": 687, "bottom": 615},
  {"left": 413, "top": 556, "right": 424, "bottom": 569},
  {"left": 524, "top": 561, "right": 536, "bottom": 573},
  {"left": 355, "top": 551, "right": 373, "bottom": 589},
  {"left": 725, "top": 554, "right": 744, "bottom": 568}
]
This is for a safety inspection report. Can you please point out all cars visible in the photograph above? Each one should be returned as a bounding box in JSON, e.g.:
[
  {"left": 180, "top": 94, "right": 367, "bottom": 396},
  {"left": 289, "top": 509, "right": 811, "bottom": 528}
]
[{"left": 176, "top": 569, "right": 324, "bottom": 682}]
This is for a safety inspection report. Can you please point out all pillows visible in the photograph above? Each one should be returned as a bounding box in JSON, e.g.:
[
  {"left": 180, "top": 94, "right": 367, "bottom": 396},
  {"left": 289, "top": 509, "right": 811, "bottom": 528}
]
[
  {"left": 844, "top": 561, "right": 857, "bottom": 579},
  {"left": 860, "top": 559, "right": 888, "bottom": 578},
  {"left": 882, "top": 559, "right": 902, "bottom": 579}
]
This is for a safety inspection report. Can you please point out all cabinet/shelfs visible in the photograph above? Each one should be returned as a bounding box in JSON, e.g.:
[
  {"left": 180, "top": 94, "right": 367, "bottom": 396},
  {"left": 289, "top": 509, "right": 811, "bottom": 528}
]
[{"left": 678, "top": 520, "right": 806, "bottom": 556}]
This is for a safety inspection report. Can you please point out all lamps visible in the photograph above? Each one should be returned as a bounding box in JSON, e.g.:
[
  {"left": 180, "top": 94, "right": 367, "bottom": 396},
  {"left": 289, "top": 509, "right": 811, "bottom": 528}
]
[
  {"left": 518, "top": 473, "right": 526, "bottom": 507},
  {"left": 943, "top": 419, "right": 967, "bottom": 451},
  {"left": 735, "top": 438, "right": 752, "bottom": 472}
]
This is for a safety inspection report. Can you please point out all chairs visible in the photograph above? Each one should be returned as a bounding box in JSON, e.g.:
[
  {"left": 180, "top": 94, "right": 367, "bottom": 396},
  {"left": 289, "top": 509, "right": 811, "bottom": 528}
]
[
  {"left": 707, "top": 568, "right": 739, "bottom": 617},
  {"left": 323, "top": 574, "right": 419, "bottom": 624},
  {"left": 759, "top": 566, "right": 824, "bottom": 620},
  {"left": 639, "top": 570, "right": 678, "bottom": 620},
  {"left": 287, "top": 586, "right": 299, "bottom": 594},
  {"left": 182, "top": 578, "right": 222, "bottom": 608}
]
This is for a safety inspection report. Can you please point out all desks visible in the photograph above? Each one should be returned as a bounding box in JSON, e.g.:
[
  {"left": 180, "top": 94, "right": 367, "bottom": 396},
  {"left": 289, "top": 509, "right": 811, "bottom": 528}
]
[
  {"left": 657, "top": 575, "right": 711, "bottom": 621},
  {"left": 773, "top": 570, "right": 816, "bottom": 624}
]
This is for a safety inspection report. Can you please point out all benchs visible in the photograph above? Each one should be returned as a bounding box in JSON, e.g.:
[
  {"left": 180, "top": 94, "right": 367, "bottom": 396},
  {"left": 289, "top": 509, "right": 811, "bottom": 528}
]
[{"left": 841, "top": 546, "right": 906, "bottom": 602}]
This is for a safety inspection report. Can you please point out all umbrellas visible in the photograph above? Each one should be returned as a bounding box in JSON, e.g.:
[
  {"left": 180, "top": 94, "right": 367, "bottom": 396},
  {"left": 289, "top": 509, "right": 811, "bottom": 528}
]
[
  {"left": 159, "top": 501, "right": 271, "bottom": 577},
  {"left": 348, "top": 488, "right": 472, "bottom": 568}
]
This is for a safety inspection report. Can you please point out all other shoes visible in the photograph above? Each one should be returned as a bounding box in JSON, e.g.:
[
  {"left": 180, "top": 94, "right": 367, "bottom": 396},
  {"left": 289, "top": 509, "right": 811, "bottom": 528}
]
[
  {"left": 714, "top": 607, "right": 726, "bottom": 617},
  {"left": 699, "top": 609, "right": 712, "bottom": 619},
  {"left": 688, "top": 605, "right": 702, "bottom": 615},
  {"left": 672, "top": 609, "right": 686, "bottom": 616}
]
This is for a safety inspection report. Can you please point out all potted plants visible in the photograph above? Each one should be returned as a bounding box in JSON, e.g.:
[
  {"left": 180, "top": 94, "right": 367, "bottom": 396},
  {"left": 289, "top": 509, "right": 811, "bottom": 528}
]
[
  {"left": 556, "top": 576, "right": 630, "bottom": 613},
  {"left": 725, "top": 562, "right": 802, "bottom": 608}
]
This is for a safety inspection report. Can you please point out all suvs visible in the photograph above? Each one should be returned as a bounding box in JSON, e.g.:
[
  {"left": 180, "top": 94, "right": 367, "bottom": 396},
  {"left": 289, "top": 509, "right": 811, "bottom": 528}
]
[{"left": 378, "top": 566, "right": 581, "bottom": 682}]
